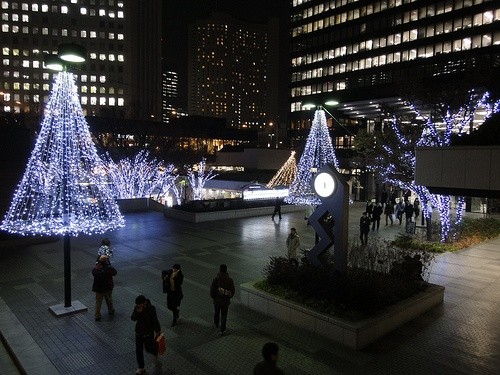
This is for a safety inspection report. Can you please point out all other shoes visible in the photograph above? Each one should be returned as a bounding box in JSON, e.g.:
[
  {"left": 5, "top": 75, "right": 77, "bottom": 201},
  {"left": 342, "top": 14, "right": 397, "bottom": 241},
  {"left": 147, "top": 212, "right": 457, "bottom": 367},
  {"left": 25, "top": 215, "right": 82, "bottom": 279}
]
[
  {"left": 109, "top": 309, "right": 115, "bottom": 315},
  {"left": 171, "top": 321, "right": 177, "bottom": 326},
  {"left": 136, "top": 368, "right": 144, "bottom": 374},
  {"left": 176, "top": 310, "right": 179, "bottom": 318},
  {"left": 220, "top": 330, "right": 224, "bottom": 335},
  {"left": 95, "top": 316, "right": 101, "bottom": 321}
]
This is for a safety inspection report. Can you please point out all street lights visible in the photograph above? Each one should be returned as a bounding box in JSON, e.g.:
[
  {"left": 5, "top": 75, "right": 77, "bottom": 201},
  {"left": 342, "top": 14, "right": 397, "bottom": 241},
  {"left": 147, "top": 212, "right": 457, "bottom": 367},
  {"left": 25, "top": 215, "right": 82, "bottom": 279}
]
[
  {"left": 43, "top": 43, "right": 89, "bottom": 307},
  {"left": 301, "top": 97, "right": 341, "bottom": 219}
]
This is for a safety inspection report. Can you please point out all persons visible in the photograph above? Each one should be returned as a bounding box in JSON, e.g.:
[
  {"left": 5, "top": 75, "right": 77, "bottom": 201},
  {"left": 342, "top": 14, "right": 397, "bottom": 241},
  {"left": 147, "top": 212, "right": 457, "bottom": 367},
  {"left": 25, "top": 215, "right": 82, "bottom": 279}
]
[
  {"left": 161, "top": 264, "right": 184, "bottom": 326},
  {"left": 286, "top": 227, "right": 301, "bottom": 260},
  {"left": 272, "top": 197, "right": 282, "bottom": 220},
  {"left": 96, "top": 238, "right": 112, "bottom": 265},
  {"left": 210, "top": 264, "right": 235, "bottom": 334},
  {"left": 360, "top": 189, "right": 421, "bottom": 246},
  {"left": 131, "top": 295, "right": 161, "bottom": 374},
  {"left": 92, "top": 255, "right": 117, "bottom": 321},
  {"left": 252, "top": 342, "right": 284, "bottom": 375},
  {"left": 305, "top": 205, "right": 314, "bottom": 226}
]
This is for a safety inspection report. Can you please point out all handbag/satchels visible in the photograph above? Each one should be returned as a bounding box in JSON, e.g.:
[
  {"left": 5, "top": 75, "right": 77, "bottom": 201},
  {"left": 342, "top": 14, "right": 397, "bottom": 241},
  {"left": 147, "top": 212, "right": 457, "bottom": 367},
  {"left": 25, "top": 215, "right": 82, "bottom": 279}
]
[
  {"left": 163, "top": 278, "right": 171, "bottom": 293},
  {"left": 154, "top": 332, "right": 165, "bottom": 353},
  {"left": 371, "top": 216, "right": 374, "bottom": 222}
]
